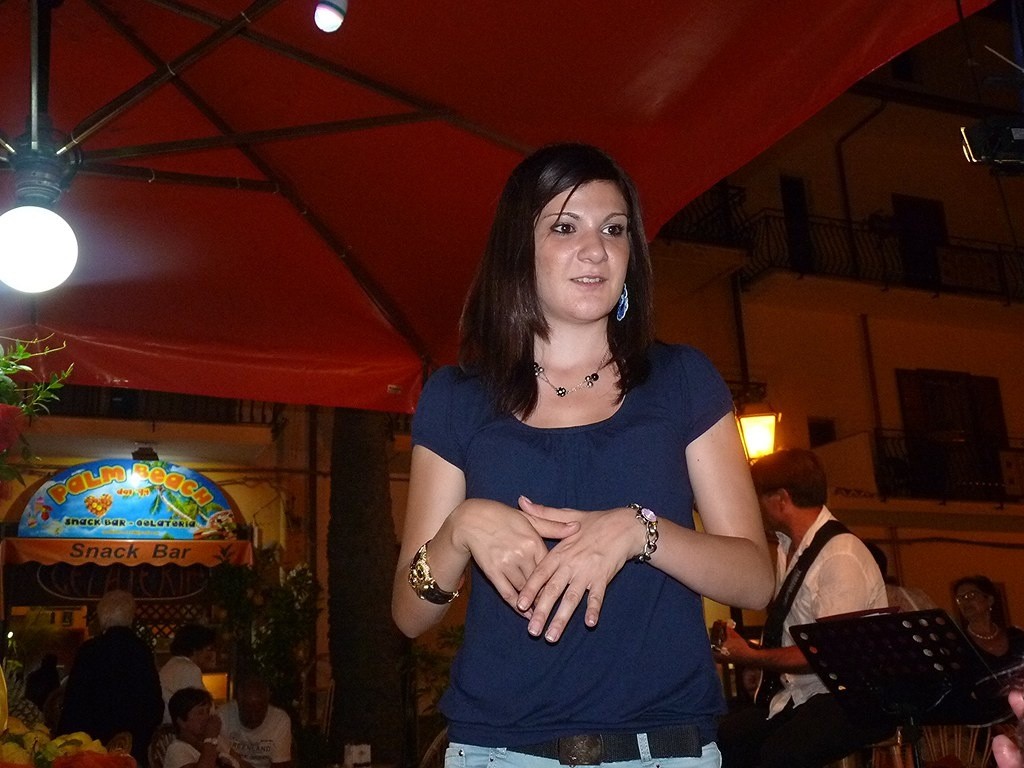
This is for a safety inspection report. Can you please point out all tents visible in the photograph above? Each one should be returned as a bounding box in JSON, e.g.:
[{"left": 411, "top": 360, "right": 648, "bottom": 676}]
[{"left": 0, "top": 1, "right": 996, "bottom": 413}]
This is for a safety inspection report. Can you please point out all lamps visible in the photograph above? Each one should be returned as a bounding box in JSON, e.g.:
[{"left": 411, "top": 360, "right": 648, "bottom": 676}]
[
  {"left": 959, "top": 122, "right": 1024, "bottom": 176},
  {"left": 132, "top": 447, "right": 159, "bottom": 461}
]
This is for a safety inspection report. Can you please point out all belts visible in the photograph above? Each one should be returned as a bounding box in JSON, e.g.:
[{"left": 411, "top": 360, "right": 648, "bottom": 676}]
[{"left": 506, "top": 726, "right": 702, "bottom": 767}]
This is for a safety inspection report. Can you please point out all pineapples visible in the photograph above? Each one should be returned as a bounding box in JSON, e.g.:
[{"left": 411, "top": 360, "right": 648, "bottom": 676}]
[{"left": 3, "top": 664, "right": 46, "bottom": 729}]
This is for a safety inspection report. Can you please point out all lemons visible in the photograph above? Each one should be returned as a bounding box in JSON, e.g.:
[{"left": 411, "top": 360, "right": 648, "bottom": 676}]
[{"left": 0, "top": 719, "right": 107, "bottom": 765}]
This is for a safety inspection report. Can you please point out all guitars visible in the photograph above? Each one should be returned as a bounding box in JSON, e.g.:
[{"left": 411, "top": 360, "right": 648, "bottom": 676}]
[{"left": 709, "top": 617, "right": 783, "bottom": 708}]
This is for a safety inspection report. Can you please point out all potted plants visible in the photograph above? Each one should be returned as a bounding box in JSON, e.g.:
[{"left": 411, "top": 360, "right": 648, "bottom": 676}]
[{"left": 0, "top": 334, "right": 75, "bottom": 488}]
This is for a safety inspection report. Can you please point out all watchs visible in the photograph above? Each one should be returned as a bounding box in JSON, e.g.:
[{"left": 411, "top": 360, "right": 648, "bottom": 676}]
[{"left": 626, "top": 502, "right": 658, "bottom": 561}]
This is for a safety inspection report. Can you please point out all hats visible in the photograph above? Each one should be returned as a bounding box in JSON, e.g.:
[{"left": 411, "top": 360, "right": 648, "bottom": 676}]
[{"left": 169, "top": 623, "right": 216, "bottom": 657}]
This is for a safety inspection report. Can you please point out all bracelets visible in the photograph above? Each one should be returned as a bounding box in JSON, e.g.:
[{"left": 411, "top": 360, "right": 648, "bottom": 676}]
[
  {"left": 407, "top": 539, "right": 466, "bottom": 604},
  {"left": 202, "top": 738, "right": 218, "bottom": 745}
]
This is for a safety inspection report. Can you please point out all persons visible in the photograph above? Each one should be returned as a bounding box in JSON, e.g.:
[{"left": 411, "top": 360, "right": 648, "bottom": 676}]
[
  {"left": 390, "top": 141, "right": 774, "bottom": 768},
  {"left": 864, "top": 541, "right": 937, "bottom": 614},
  {"left": 710, "top": 449, "right": 888, "bottom": 768},
  {"left": 56, "top": 589, "right": 165, "bottom": 768},
  {"left": 953, "top": 576, "right": 1024, "bottom": 768},
  {"left": 158, "top": 622, "right": 296, "bottom": 768},
  {"left": 26, "top": 654, "right": 60, "bottom": 711}
]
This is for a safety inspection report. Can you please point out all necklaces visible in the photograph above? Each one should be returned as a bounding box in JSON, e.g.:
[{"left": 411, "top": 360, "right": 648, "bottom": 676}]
[
  {"left": 533, "top": 349, "right": 617, "bottom": 396},
  {"left": 968, "top": 622, "right": 999, "bottom": 640}
]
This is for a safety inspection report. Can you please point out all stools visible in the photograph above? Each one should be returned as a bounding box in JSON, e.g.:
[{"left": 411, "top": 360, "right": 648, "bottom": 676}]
[{"left": 832, "top": 723, "right": 916, "bottom": 768}]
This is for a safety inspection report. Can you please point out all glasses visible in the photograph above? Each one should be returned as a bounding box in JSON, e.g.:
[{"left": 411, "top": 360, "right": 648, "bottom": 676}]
[{"left": 954, "top": 590, "right": 988, "bottom": 605}]
